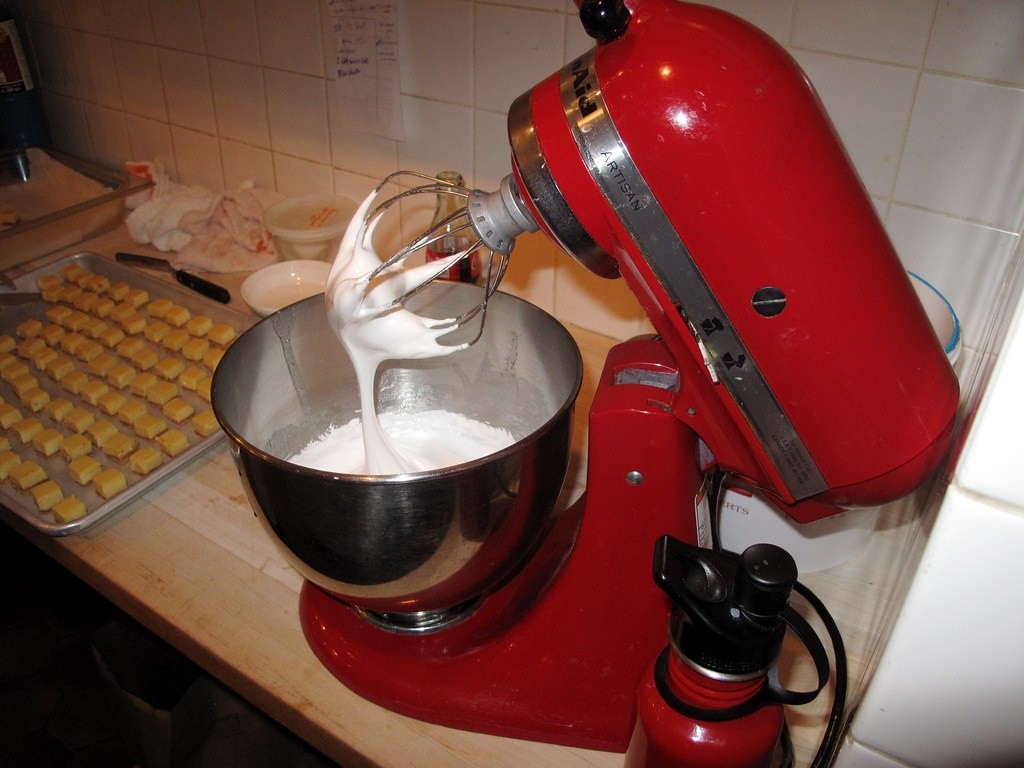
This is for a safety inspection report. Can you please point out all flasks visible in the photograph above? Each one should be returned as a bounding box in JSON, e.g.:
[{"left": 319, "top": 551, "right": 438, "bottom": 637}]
[{"left": 640, "top": 535, "right": 830, "bottom": 768}]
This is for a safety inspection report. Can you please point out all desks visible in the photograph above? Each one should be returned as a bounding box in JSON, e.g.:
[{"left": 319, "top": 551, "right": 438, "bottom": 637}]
[{"left": 0, "top": 189, "right": 854, "bottom": 768}]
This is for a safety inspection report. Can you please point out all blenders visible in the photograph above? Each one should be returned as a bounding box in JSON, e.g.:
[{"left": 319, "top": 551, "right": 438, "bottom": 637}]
[{"left": 209, "top": 4, "right": 960, "bottom": 767}]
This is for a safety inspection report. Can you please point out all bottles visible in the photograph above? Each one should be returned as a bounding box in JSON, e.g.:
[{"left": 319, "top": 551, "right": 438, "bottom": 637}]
[{"left": 425, "top": 171, "right": 485, "bottom": 287}]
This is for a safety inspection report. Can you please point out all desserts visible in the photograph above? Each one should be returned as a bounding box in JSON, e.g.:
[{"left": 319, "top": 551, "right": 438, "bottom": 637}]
[{"left": 0, "top": 264, "right": 235, "bottom": 525}]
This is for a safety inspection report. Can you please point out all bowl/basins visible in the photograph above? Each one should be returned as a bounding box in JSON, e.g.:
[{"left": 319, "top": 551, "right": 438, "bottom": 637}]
[
  {"left": 265, "top": 193, "right": 360, "bottom": 264},
  {"left": 240, "top": 259, "right": 332, "bottom": 317}
]
